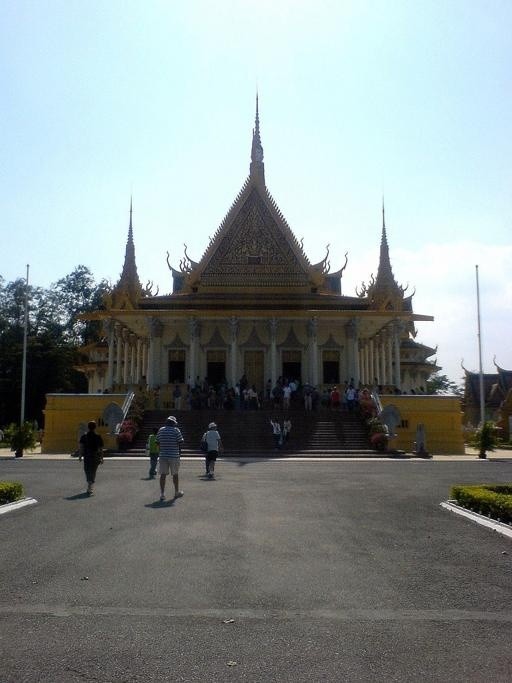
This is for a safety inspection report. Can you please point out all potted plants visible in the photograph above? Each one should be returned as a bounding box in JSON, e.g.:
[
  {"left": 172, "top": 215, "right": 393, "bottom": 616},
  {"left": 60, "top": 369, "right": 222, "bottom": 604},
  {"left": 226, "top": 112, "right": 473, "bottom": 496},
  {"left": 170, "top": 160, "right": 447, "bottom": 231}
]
[
  {"left": 2, "top": 418, "right": 39, "bottom": 458},
  {"left": 469, "top": 420, "right": 503, "bottom": 458}
]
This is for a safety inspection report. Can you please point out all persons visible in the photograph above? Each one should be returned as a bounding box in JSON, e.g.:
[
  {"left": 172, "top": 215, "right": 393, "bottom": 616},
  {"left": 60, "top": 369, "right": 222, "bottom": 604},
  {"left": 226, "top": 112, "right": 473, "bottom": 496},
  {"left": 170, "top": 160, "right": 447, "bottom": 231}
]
[
  {"left": 158, "top": 416, "right": 184, "bottom": 502},
  {"left": 183, "top": 373, "right": 368, "bottom": 413},
  {"left": 269, "top": 419, "right": 282, "bottom": 446},
  {"left": 201, "top": 422, "right": 222, "bottom": 479},
  {"left": 283, "top": 416, "right": 292, "bottom": 441},
  {"left": 78, "top": 421, "right": 105, "bottom": 494},
  {"left": 147, "top": 428, "right": 160, "bottom": 475}
]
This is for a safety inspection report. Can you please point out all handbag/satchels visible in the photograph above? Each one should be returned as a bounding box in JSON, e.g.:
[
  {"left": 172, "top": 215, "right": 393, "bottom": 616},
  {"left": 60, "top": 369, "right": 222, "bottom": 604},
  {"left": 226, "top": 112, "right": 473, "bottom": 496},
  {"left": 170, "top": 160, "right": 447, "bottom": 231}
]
[{"left": 201, "top": 433, "right": 208, "bottom": 450}]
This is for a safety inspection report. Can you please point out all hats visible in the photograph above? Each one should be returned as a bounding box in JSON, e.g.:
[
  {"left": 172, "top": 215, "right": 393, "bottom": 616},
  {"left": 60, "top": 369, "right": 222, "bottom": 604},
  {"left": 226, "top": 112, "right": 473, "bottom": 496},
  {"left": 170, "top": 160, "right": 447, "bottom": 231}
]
[
  {"left": 167, "top": 415, "right": 178, "bottom": 424},
  {"left": 208, "top": 422, "right": 218, "bottom": 428}
]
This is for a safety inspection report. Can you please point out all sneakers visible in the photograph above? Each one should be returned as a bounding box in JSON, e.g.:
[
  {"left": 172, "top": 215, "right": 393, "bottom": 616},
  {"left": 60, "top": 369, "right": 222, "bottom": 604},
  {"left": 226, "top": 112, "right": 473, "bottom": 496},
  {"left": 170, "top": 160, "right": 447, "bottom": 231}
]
[
  {"left": 160, "top": 494, "right": 166, "bottom": 501},
  {"left": 174, "top": 491, "right": 184, "bottom": 498}
]
